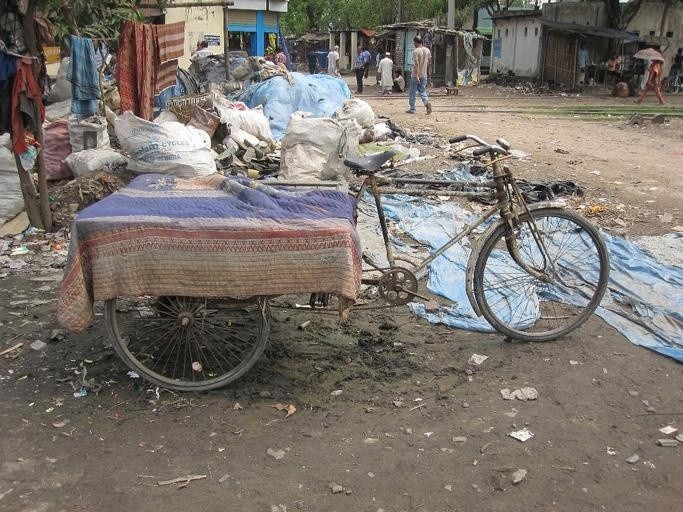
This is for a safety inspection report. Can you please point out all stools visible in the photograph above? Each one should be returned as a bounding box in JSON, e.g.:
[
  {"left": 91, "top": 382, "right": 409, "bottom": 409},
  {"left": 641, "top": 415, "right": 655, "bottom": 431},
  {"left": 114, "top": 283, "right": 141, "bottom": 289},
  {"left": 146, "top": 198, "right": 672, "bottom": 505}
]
[{"left": 446, "top": 86, "right": 459, "bottom": 96}]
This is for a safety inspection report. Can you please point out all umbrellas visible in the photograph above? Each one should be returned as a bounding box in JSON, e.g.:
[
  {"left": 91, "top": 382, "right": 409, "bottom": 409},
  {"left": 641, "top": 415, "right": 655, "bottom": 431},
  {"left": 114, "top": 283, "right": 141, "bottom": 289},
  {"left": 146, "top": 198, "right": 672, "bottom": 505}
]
[{"left": 632, "top": 47, "right": 664, "bottom": 61}]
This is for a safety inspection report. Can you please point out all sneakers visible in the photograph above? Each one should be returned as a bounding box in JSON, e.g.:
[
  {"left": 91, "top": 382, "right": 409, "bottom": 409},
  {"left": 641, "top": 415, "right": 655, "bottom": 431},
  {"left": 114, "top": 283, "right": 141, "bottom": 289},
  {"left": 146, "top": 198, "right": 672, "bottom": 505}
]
[{"left": 426, "top": 103, "right": 432, "bottom": 114}]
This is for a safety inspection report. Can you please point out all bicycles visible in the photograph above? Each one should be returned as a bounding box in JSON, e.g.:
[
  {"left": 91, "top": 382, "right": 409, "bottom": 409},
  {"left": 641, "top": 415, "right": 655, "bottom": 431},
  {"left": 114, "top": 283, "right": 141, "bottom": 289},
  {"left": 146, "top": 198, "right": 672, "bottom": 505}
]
[{"left": 666, "top": 64, "right": 682, "bottom": 96}]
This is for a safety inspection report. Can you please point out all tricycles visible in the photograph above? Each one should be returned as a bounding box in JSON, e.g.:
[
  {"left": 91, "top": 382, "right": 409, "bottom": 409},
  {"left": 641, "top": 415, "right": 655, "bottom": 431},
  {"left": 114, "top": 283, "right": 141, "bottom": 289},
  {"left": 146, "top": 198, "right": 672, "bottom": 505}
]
[{"left": 67, "top": 129, "right": 613, "bottom": 396}]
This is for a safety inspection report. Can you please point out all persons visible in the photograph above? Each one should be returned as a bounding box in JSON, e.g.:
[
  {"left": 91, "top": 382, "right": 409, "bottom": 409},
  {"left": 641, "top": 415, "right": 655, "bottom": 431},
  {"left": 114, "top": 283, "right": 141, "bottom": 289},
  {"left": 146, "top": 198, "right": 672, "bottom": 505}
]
[
  {"left": 363, "top": 47, "right": 371, "bottom": 78},
  {"left": 274, "top": 47, "right": 286, "bottom": 64},
  {"left": 327, "top": 45, "right": 340, "bottom": 77},
  {"left": 608, "top": 52, "right": 633, "bottom": 83},
  {"left": 375, "top": 49, "right": 405, "bottom": 95},
  {"left": 355, "top": 46, "right": 367, "bottom": 94},
  {"left": 307, "top": 51, "right": 319, "bottom": 74},
  {"left": 406, "top": 36, "right": 432, "bottom": 115},
  {"left": 675, "top": 48, "right": 683, "bottom": 68},
  {"left": 633, "top": 60, "right": 665, "bottom": 106}
]
